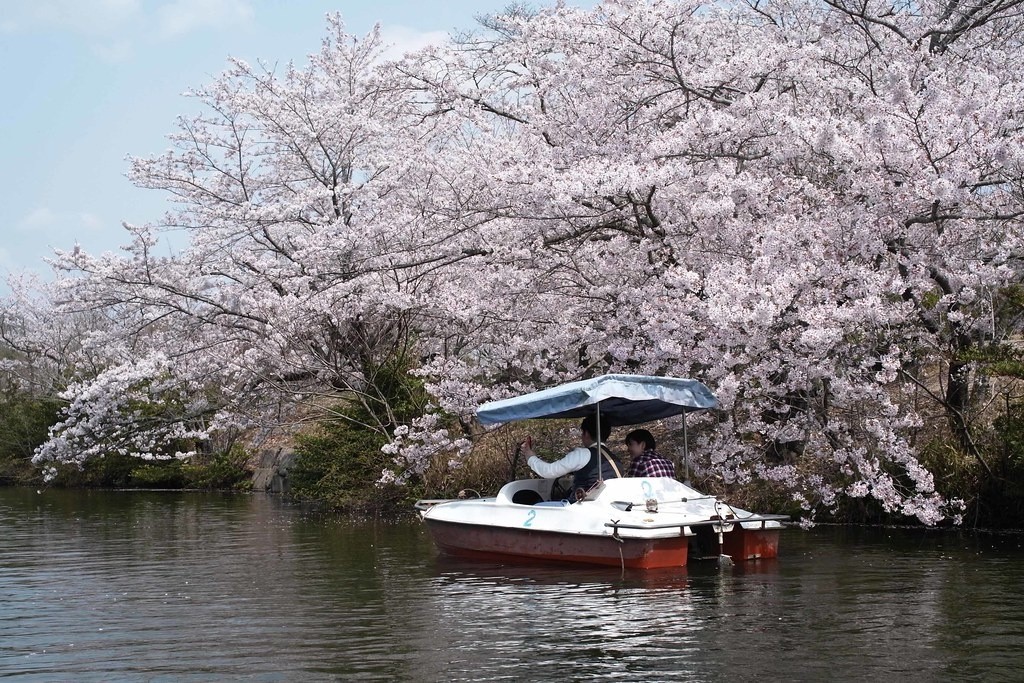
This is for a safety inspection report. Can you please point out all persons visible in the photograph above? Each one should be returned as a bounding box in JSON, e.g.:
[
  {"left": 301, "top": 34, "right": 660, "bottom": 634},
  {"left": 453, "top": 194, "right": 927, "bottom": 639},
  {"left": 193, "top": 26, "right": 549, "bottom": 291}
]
[
  {"left": 625, "top": 428, "right": 678, "bottom": 482},
  {"left": 521, "top": 412, "right": 625, "bottom": 507}
]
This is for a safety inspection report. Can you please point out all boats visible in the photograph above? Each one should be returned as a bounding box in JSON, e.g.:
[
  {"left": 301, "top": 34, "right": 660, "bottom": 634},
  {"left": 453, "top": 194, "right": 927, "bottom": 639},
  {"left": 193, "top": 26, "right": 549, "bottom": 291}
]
[{"left": 413, "top": 372, "right": 793, "bottom": 569}]
